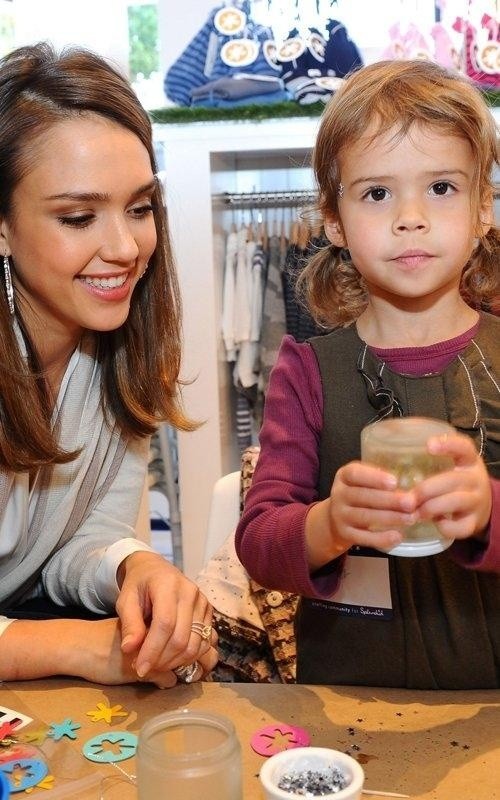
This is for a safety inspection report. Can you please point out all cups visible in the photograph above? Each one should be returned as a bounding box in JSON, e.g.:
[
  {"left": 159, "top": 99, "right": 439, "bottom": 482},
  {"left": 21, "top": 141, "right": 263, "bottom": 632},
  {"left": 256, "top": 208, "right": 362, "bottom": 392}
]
[
  {"left": 360, "top": 416, "right": 459, "bottom": 559},
  {"left": 258, "top": 746, "right": 366, "bottom": 800},
  {"left": 133, "top": 708, "right": 244, "bottom": 799}
]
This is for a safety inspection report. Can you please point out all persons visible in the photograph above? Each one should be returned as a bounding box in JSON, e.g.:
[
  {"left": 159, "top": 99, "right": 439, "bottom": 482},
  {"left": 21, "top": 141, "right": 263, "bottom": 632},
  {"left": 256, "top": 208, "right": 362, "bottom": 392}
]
[
  {"left": 0, "top": 41, "right": 223, "bottom": 692},
  {"left": 231, "top": 57, "right": 498, "bottom": 690}
]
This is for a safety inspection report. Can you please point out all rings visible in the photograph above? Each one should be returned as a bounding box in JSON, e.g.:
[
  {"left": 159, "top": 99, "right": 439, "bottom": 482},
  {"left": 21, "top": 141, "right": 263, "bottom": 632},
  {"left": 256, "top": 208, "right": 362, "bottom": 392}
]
[
  {"left": 191, "top": 620, "right": 214, "bottom": 642},
  {"left": 176, "top": 659, "right": 199, "bottom": 685}
]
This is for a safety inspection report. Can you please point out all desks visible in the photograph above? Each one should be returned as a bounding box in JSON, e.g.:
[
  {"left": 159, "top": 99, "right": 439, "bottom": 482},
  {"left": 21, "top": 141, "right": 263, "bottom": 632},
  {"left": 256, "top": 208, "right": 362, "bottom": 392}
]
[{"left": 2, "top": 673, "right": 499, "bottom": 800}]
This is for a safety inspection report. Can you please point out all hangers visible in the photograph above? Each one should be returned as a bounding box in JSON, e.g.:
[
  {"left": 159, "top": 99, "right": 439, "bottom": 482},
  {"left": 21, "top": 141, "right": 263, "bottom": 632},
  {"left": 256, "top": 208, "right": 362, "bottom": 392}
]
[{"left": 225, "top": 185, "right": 324, "bottom": 255}]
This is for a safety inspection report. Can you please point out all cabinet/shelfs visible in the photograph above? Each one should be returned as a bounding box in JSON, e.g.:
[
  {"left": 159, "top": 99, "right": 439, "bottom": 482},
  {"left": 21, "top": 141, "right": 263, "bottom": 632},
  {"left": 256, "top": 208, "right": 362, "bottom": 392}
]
[{"left": 140, "top": 105, "right": 499, "bottom": 593}]
